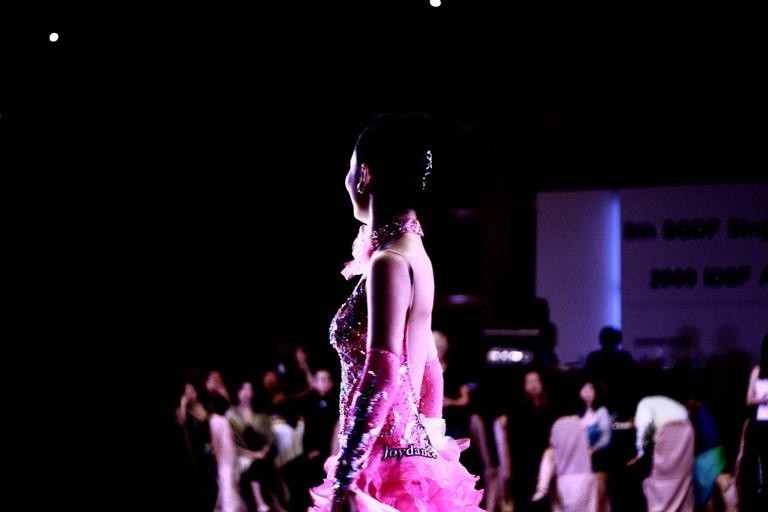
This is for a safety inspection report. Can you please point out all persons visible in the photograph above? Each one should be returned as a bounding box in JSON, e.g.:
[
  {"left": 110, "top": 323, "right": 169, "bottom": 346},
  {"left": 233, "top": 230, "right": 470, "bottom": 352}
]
[
  {"left": 179, "top": 344, "right": 340, "bottom": 512},
  {"left": 431, "top": 325, "right": 768, "bottom": 512},
  {"left": 308, "top": 114, "right": 487, "bottom": 512}
]
